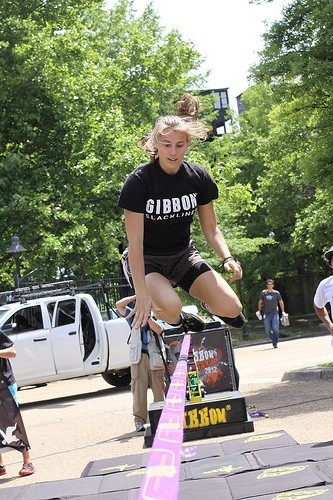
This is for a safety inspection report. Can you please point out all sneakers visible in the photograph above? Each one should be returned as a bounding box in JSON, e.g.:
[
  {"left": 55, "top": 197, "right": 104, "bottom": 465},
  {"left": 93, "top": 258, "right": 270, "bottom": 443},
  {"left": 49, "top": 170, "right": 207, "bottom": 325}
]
[
  {"left": 19, "top": 462, "right": 35, "bottom": 476},
  {"left": 0, "top": 465, "right": 6, "bottom": 475},
  {"left": 172, "top": 310, "right": 207, "bottom": 332},
  {"left": 201, "top": 302, "right": 247, "bottom": 330},
  {"left": 135, "top": 420, "right": 145, "bottom": 432}
]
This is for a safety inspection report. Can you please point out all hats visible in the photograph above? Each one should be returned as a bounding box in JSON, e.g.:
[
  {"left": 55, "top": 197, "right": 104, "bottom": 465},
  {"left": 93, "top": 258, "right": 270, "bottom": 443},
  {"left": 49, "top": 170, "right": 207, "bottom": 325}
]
[{"left": 324, "top": 246, "right": 333, "bottom": 260}]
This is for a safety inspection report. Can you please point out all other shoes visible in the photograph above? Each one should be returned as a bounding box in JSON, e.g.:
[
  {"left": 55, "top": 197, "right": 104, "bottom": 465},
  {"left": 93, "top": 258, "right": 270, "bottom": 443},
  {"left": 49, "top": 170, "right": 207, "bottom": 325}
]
[{"left": 274, "top": 344, "right": 277, "bottom": 348}]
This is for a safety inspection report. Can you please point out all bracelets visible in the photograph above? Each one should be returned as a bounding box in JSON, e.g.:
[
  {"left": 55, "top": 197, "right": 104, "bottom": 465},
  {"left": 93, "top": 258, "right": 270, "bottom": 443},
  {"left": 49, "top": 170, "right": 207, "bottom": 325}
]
[
  {"left": 147, "top": 316, "right": 150, "bottom": 319},
  {"left": 218, "top": 256, "right": 237, "bottom": 267}
]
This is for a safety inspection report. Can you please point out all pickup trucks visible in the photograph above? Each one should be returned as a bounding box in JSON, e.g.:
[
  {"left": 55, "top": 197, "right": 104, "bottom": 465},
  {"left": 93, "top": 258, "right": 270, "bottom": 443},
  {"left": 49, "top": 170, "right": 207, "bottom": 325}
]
[{"left": 0, "top": 281, "right": 198, "bottom": 387}]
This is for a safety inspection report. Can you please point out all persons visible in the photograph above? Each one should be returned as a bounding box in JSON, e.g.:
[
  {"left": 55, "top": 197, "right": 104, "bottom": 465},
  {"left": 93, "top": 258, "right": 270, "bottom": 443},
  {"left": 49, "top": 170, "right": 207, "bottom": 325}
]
[
  {"left": 116, "top": 295, "right": 166, "bottom": 432},
  {"left": 118, "top": 93, "right": 246, "bottom": 332},
  {"left": 0, "top": 330, "right": 36, "bottom": 476},
  {"left": 256, "top": 279, "right": 290, "bottom": 348},
  {"left": 314, "top": 245, "right": 333, "bottom": 350}
]
